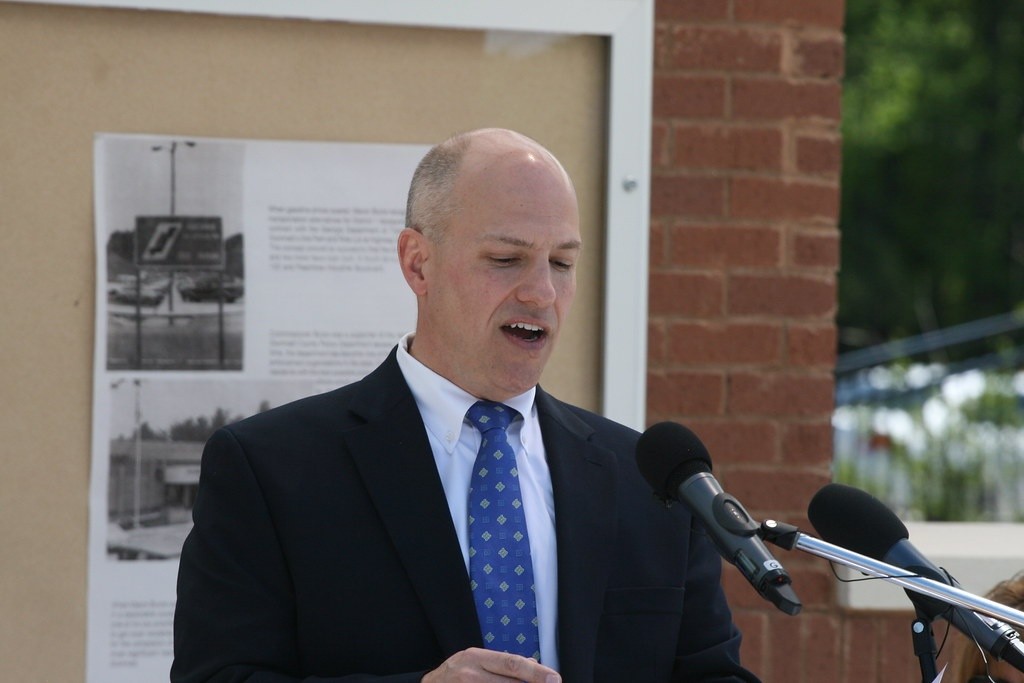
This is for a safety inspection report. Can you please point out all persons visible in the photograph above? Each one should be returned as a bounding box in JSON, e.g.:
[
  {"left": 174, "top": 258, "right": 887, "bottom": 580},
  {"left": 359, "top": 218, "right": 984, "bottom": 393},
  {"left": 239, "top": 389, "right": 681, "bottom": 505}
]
[{"left": 171, "top": 126, "right": 771, "bottom": 683}]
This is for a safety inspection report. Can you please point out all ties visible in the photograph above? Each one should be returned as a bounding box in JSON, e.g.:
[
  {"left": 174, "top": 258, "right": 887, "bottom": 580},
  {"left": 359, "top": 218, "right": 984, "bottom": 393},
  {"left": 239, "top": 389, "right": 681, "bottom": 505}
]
[{"left": 465, "top": 401, "right": 542, "bottom": 669}]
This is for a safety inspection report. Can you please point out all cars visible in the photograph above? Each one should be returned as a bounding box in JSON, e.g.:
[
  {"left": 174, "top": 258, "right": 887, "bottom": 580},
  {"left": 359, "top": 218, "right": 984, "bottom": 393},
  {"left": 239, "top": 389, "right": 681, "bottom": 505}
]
[{"left": 832, "top": 365, "right": 1023, "bottom": 503}]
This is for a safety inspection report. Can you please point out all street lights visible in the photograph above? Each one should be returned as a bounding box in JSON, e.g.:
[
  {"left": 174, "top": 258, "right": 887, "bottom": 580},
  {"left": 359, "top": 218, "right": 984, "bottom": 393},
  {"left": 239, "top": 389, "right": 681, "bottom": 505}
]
[
  {"left": 150, "top": 140, "right": 195, "bottom": 311},
  {"left": 109, "top": 378, "right": 141, "bottom": 528}
]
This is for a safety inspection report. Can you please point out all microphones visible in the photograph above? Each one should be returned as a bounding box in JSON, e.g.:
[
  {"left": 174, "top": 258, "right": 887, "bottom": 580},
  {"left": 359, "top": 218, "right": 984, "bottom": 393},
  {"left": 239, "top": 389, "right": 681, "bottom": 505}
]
[
  {"left": 635, "top": 421, "right": 804, "bottom": 617},
  {"left": 806, "top": 483, "right": 1023, "bottom": 674}
]
